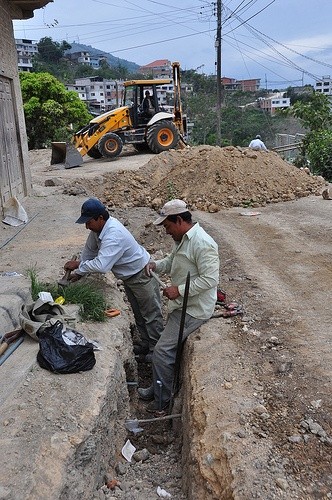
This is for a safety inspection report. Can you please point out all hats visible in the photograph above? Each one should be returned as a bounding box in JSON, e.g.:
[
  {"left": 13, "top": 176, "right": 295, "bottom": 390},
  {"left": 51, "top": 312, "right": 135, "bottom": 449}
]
[
  {"left": 153, "top": 199, "right": 188, "bottom": 225},
  {"left": 75, "top": 198, "right": 105, "bottom": 224}
]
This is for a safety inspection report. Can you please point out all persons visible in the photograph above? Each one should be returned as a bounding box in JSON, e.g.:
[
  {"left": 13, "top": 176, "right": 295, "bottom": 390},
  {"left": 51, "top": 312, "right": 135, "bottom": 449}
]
[
  {"left": 143, "top": 90, "right": 154, "bottom": 117},
  {"left": 136, "top": 199, "right": 219, "bottom": 414},
  {"left": 249, "top": 135, "right": 268, "bottom": 153},
  {"left": 64, "top": 199, "right": 164, "bottom": 363}
]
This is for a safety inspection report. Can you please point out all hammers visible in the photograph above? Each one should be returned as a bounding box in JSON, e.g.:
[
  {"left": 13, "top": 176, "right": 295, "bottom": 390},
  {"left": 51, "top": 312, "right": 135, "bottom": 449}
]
[{"left": 57, "top": 253, "right": 78, "bottom": 287}]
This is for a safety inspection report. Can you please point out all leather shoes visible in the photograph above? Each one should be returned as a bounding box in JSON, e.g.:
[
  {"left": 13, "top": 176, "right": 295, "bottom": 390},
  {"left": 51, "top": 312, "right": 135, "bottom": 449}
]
[{"left": 138, "top": 385, "right": 154, "bottom": 399}]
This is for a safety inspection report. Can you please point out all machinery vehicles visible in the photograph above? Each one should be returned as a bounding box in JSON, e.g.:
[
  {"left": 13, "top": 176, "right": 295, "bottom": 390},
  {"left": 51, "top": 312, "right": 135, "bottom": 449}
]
[{"left": 49, "top": 62, "right": 183, "bottom": 169}]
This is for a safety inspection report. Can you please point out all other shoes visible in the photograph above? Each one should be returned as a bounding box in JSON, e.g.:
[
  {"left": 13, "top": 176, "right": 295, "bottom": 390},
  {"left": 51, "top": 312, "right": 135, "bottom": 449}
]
[{"left": 146, "top": 400, "right": 169, "bottom": 410}]
